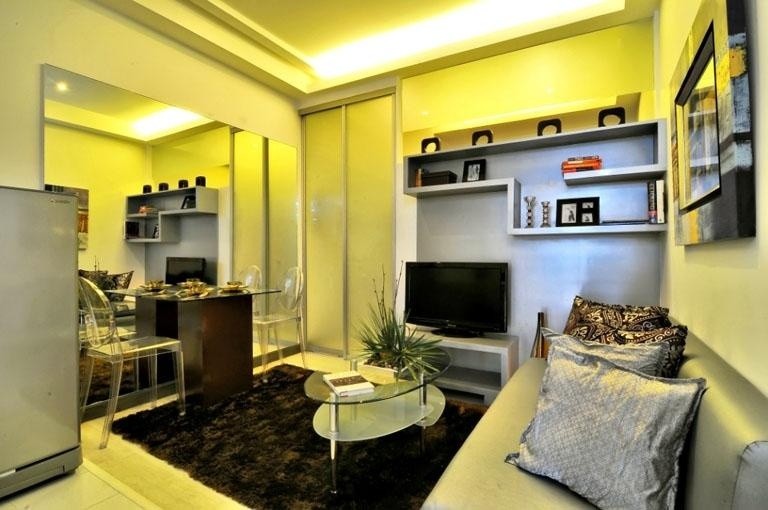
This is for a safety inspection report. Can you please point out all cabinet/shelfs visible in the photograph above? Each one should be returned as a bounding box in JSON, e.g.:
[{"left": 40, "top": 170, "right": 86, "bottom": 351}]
[
  {"left": 402, "top": 118, "right": 667, "bottom": 235},
  {"left": 400, "top": 329, "right": 519, "bottom": 406},
  {"left": 123, "top": 187, "right": 218, "bottom": 244}
]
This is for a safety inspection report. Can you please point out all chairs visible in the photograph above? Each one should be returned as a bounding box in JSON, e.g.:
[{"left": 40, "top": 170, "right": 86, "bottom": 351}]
[
  {"left": 79, "top": 276, "right": 187, "bottom": 449},
  {"left": 240, "top": 265, "right": 265, "bottom": 319},
  {"left": 253, "top": 266, "right": 308, "bottom": 383}
]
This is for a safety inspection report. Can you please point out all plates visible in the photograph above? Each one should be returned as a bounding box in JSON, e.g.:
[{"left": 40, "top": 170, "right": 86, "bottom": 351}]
[
  {"left": 219, "top": 285, "right": 249, "bottom": 293},
  {"left": 141, "top": 284, "right": 173, "bottom": 291},
  {"left": 177, "top": 282, "right": 206, "bottom": 288}
]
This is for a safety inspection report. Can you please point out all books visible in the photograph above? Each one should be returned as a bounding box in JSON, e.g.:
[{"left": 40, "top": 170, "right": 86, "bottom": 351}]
[
  {"left": 603, "top": 218, "right": 648, "bottom": 224},
  {"left": 561, "top": 155, "right": 602, "bottom": 174},
  {"left": 647, "top": 179, "right": 665, "bottom": 225},
  {"left": 322, "top": 371, "right": 375, "bottom": 397}
]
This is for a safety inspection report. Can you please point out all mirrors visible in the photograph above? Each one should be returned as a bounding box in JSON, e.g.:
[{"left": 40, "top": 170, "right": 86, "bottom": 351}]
[{"left": 39, "top": 62, "right": 299, "bottom": 421}]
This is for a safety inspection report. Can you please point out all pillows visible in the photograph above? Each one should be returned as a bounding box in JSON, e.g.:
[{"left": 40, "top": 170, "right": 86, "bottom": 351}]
[
  {"left": 78, "top": 269, "right": 108, "bottom": 289},
  {"left": 539, "top": 329, "right": 669, "bottom": 380},
  {"left": 571, "top": 320, "right": 688, "bottom": 377},
  {"left": 107, "top": 271, "right": 133, "bottom": 300},
  {"left": 564, "top": 295, "right": 669, "bottom": 335},
  {"left": 504, "top": 340, "right": 707, "bottom": 510}
]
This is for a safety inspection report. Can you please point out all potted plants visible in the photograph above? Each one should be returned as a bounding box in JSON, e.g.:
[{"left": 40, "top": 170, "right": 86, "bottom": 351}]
[{"left": 354, "top": 258, "right": 446, "bottom": 384}]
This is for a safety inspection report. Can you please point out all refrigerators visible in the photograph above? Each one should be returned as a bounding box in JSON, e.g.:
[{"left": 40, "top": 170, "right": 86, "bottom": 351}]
[{"left": 0, "top": 185, "right": 93, "bottom": 488}]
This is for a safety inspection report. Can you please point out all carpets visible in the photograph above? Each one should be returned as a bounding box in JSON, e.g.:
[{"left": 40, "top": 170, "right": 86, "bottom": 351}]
[
  {"left": 80, "top": 338, "right": 176, "bottom": 406},
  {"left": 112, "top": 364, "right": 484, "bottom": 510}
]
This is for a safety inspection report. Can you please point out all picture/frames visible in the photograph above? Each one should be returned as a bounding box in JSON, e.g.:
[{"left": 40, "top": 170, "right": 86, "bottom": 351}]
[
  {"left": 672, "top": 1, "right": 757, "bottom": 245},
  {"left": 555, "top": 196, "right": 600, "bottom": 225},
  {"left": 462, "top": 158, "right": 486, "bottom": 182}
]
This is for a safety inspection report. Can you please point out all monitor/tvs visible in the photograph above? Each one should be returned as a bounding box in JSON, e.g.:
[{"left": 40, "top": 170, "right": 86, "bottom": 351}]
[
  {"left": 403, "top": 261, "right": 509, "bottom": 338},
  {"left": 165, "top": 257, "right": 207, "bottom": 287}
]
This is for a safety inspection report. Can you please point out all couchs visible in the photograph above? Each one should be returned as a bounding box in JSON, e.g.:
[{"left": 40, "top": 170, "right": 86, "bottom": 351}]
[{"left": 421, "top": 318, "right": 768, "bottom": 510}]
